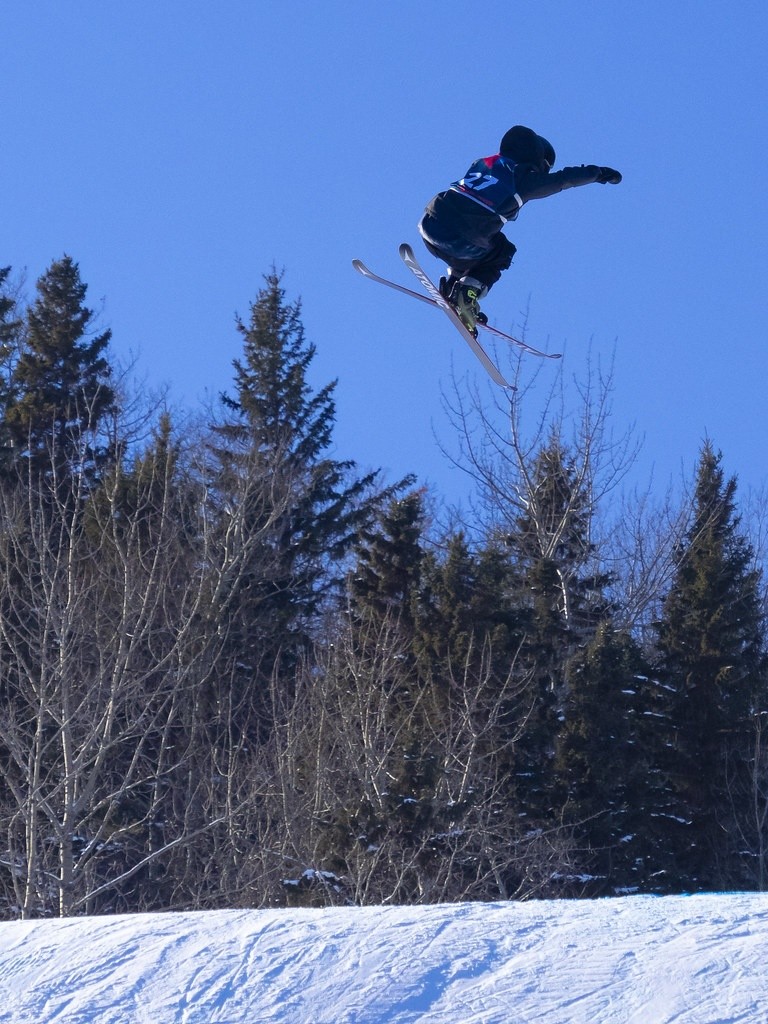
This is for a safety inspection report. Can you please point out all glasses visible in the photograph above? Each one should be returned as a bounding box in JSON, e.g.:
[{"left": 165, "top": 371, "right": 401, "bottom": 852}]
[{"left": 543, "top": 159, "right": 554, "bottom": 170}]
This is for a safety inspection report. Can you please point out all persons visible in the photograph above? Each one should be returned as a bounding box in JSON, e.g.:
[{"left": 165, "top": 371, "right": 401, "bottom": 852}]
[{"left": 419, "top": 126, "right": 622, "bottom": 339}]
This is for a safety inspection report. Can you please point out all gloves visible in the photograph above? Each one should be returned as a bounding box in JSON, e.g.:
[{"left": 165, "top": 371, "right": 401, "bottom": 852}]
[{"left": 595, "top": 167, "right": 622, "bottom": 184}]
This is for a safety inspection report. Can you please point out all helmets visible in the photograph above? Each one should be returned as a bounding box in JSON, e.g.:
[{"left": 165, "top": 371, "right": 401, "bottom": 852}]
[{"left": 538, "top": 136, "right": 555, "bottom": 174}]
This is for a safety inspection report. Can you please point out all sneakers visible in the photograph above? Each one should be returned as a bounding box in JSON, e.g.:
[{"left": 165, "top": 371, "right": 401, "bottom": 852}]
[
  {"left": 446, "top": 280, "right": 479, "bottom": 340},
  {"left": 439, "top": 276, "right": 488, "bottom": 324}
]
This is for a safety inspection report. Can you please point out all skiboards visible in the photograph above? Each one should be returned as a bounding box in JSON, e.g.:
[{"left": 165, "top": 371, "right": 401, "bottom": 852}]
[{"left": 352, "top": 243, "right": 562, "bottom": 392}]
